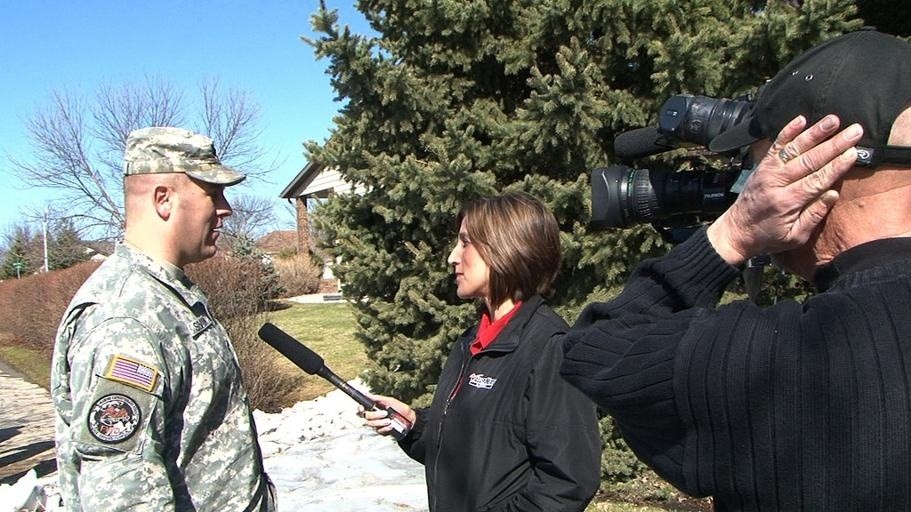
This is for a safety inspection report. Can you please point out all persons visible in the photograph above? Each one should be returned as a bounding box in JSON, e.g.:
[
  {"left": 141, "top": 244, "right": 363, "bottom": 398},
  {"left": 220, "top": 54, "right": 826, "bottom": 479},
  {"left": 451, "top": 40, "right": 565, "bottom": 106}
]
[
  {"left": 562, "top": 29, "right": 910, "bottom": 511},
  {"left": 50, "top": 124, "right": 284, "bottom": 511},
  {"left": 357, "top": 188, "right": 605, "bottom": 512}
]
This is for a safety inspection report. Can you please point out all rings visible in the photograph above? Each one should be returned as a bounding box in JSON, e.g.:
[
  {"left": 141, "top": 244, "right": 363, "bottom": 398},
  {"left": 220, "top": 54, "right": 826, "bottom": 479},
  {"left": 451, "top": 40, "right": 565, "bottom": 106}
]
[{"left": 778, "top": 148, "right": 792, "bottom": 164}]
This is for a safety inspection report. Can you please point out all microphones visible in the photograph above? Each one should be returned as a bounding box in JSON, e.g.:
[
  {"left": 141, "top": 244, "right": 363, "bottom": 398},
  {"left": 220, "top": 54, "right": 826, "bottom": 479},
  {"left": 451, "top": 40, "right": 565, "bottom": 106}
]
[
  {"left": 614, "top": 127, "right": 676, "bottom": 160},
  {"left": 258, "top": 323, "right": 387, "bottom": 413}
]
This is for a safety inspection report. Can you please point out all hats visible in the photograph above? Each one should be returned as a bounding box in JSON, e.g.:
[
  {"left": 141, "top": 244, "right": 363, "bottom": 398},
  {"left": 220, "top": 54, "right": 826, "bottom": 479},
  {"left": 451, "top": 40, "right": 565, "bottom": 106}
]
[
  {"left": 708, "top": 31, "right": 911, "bottom": 171},
  {"left": 122, "top": 128, "right": 247, "bottom": 186}
]
